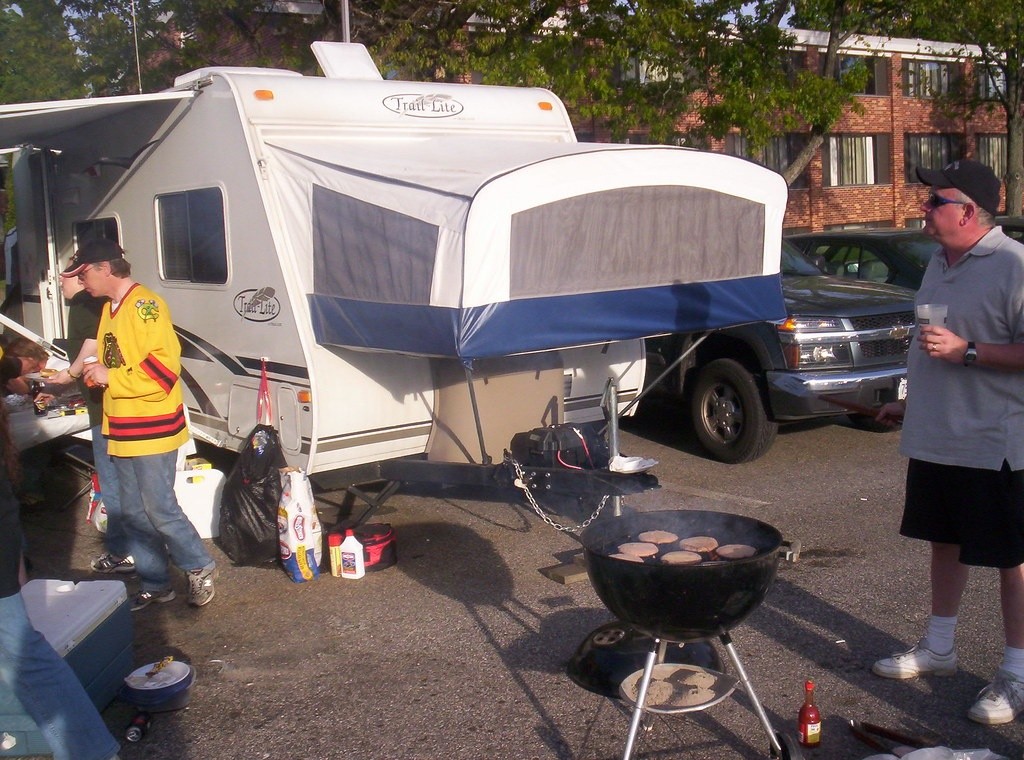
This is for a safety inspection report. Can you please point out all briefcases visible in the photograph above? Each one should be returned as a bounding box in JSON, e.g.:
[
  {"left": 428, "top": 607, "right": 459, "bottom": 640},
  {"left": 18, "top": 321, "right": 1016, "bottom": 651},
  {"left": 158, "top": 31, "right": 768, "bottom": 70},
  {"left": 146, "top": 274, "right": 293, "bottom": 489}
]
[{"left": 524, "top": 422, "right": 609, "bottom": 469}]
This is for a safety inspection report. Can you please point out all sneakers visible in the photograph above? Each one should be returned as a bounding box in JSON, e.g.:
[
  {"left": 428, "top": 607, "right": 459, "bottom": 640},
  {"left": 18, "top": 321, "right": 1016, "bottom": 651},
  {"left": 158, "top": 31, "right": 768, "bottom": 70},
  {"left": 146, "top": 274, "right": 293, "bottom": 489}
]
[
  {"left": 873, "top": 633, "right": 957, "bottom": 678},
  {"left": 91, "top": 552, "right": 135, "bottom": 573},
  {"left": 129, "top": 588, "right": 176, "bottom": 611},
  {"left": 967, "top": 666, "right": 1024, "bottom": 724},
  {"left": 186, "top": 569, "right": 215, "bottom": 606}
]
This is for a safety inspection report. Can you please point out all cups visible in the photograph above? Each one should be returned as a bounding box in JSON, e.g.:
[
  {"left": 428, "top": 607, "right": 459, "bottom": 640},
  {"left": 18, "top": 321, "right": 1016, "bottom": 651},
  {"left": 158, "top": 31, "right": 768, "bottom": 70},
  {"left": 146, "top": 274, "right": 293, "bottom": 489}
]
[{"left": 917, "top": 303, "right": 949, "bottom": 337}]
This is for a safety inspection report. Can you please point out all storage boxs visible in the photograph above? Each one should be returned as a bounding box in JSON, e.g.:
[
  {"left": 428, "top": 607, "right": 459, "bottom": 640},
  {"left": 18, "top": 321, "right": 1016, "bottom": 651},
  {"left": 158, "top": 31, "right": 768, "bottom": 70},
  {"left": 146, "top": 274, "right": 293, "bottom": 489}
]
[{"left": 0, "top": 580, "right": 133, "bottom": 758}]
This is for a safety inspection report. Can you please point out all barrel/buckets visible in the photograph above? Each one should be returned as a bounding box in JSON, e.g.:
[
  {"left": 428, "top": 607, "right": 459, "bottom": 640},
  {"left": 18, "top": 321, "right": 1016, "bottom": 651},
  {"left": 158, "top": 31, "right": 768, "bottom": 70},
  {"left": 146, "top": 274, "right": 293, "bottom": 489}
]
[{"left": 119, "top": 674, "right": 192, "bottom": 714}]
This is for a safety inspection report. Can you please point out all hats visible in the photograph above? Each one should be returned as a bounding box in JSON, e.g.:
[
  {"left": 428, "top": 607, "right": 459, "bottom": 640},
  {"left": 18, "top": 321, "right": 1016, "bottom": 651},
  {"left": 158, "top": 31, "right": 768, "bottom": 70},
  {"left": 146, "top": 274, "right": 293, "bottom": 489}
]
[{"left": 60, "top": 239, "right": 123, "bottom": 278}]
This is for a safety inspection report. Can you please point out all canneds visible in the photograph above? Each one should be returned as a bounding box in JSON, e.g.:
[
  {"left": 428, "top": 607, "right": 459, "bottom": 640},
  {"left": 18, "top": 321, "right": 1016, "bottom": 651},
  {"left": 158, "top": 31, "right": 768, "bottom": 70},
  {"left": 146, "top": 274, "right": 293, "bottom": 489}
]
[
  {"left": 83, "top": 355, "right": 101, "bottom": 389},
  {"left": 125, "top": 711, "right": 149, "bottom": 741},
  {"left": 32, "top": 379, "right": 48, "bottom": 417}
]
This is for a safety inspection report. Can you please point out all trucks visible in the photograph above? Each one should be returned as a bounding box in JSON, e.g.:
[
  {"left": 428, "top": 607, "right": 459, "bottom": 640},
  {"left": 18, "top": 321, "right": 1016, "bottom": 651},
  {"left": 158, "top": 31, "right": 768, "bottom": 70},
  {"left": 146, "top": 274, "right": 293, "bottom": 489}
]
[{"left": 0, "top": 36, "right": 790, "bottom": 487}]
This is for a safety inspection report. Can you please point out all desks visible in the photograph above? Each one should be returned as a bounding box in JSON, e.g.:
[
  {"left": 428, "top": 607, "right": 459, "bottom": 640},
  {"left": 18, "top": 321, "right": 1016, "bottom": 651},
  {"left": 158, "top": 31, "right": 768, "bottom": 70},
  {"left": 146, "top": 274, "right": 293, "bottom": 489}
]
[{"left": 6, "top": 384, "right": 100, "bottom": 510}]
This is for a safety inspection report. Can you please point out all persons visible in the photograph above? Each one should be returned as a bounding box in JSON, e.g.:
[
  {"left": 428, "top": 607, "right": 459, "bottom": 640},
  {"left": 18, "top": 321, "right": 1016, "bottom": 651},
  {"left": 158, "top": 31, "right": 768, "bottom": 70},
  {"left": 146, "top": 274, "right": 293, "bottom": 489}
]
[
  {"left": 47, "top": 255, "right": 136, "bottom": 573},
  {"left": 67, "top": 217, "right": 220, "bottom": 614},
  {"left": 870, "top": 159, "right": 1024, "bottom": 727},
  {"left": 0, "top": 324, "right": 49, "bottom": 599}
]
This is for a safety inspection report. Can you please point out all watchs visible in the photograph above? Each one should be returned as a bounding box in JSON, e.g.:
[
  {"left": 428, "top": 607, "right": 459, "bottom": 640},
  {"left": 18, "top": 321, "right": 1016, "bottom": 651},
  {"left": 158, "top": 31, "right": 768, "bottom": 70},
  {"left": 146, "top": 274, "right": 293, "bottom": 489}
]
[{"left": 964, "top": 342, "right": 978, "bottom": 368}]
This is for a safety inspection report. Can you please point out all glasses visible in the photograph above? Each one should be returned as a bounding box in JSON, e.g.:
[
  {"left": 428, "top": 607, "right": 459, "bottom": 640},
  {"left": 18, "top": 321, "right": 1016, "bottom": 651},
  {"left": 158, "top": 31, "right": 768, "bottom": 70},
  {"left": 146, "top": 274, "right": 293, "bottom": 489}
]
[
  {"left": 929, "top": 191, "right": 963, "bottom": 208},
  {"left": 916, "top": 160, "right": 1001, "bottom": 217},
  {"left": 78, "top": 267, "right": 92, "bottom": 279}
]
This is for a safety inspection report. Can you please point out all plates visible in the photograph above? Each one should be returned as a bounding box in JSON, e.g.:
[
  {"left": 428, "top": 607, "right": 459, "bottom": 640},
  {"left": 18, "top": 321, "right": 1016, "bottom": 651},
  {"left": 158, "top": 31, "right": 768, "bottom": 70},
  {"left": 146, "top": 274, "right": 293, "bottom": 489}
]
[{"left": 125, "top": 661, "right": 190, "bottom": 690}]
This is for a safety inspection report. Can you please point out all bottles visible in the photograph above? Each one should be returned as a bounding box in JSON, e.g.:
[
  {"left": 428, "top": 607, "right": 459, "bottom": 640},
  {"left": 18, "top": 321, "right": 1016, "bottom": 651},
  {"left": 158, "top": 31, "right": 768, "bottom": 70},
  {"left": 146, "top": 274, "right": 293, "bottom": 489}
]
[
  {"left": 798, "top": 681, "right": 822, "bottom": 746},
  {"left": 328, "top": 534, "right": 344, "bottom": 577},
  {"left": 32, "top": 382, "right": 48, "bottom": 417},
  {"left": 340, "top": 529, "right": 366, "bottom": 579}
]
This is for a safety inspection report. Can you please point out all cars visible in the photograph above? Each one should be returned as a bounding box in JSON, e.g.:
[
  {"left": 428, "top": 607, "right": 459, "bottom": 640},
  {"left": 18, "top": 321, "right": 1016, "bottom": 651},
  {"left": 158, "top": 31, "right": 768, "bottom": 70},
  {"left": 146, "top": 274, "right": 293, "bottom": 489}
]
[
  {"left": 783, "top": 222, "right": 942, "bottom": 292},
  {"left": 636, "top": 229, "right": 920, "bottom": 466}
]
